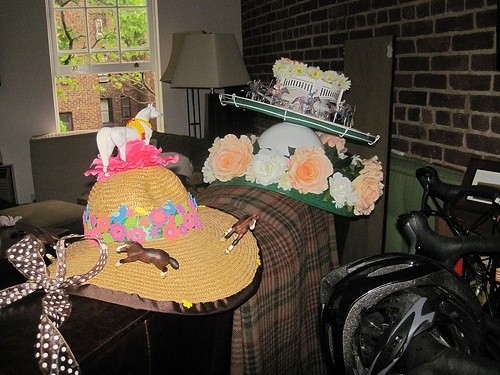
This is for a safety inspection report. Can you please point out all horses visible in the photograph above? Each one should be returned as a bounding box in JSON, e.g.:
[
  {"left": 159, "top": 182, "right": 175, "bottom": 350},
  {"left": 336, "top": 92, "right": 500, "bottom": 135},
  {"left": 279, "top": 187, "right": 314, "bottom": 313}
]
[
  {"left": 219, "top": 214, "right": 259, "bottom": 256},
  {"left": 97, "top": 103, "right": 163, "bottom": 177},
  {"left": 114, "top": 240, "right": 179, "bottom": 279}
]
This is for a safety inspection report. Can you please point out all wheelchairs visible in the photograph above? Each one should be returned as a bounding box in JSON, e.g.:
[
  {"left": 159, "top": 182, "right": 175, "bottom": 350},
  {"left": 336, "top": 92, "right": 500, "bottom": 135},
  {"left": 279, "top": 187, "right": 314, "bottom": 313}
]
[{"left": 322, "top": 167, "right": 500, "bottom": 375}]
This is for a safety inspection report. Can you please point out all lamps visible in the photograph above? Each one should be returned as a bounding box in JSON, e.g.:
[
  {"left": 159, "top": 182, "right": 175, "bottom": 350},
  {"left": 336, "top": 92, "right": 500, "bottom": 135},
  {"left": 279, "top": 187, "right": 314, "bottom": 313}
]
[{"left": 159, "top": 20, "right": 253, "bottom": 139}]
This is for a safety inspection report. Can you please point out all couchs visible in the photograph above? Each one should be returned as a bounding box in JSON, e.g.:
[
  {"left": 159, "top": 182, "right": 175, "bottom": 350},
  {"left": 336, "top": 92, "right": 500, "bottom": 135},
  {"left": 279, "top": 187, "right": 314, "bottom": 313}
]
[{"left": 1, "top": 184, "right": 338, "bottom": 375}]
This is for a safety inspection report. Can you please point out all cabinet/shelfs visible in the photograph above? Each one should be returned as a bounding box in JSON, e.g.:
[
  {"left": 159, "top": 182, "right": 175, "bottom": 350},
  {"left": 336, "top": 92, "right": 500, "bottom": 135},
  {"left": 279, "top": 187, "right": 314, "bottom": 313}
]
[{"left": 28, "top": 128, "right": 102, "bottom": 201}]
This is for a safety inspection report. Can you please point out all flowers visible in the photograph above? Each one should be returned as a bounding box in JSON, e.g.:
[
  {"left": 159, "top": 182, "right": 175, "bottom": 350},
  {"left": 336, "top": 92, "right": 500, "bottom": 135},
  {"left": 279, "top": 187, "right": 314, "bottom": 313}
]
[
  {"left": 84, "top": 138, "right": 180, "bottom": 184},
  {"left": 200, "top": 132, "right": 387, "bottom": 217},
  {"left": 270, "top": 56, "right": 352, "bottom": 93}
]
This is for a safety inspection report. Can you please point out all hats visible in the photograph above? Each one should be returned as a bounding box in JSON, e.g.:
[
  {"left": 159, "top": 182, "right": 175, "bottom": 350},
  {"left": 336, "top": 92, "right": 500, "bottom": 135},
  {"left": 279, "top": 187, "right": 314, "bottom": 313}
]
[
  {"left": 200, "top": 122, "right": 384, "bottom": 217},
  {"left": 0, "top": 103, "right": 264, "bottom": 375},
  {"left": 219, "top": 59, "right": 380, "bottom": 145}
]
